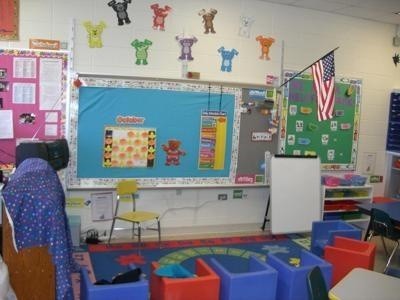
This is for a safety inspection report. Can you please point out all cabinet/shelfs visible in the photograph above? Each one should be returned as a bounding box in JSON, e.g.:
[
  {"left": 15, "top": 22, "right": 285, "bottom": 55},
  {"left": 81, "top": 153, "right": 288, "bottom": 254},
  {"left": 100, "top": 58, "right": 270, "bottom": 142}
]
[{"left": 320, "top": 183, "right": 374, "bottom": 240}]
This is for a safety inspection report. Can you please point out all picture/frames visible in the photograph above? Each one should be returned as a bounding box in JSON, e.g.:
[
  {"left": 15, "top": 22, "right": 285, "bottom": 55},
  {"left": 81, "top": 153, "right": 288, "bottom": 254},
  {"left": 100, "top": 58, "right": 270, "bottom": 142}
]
[{"left": 0, "top": 0, "right": 19, "bottom": 41}]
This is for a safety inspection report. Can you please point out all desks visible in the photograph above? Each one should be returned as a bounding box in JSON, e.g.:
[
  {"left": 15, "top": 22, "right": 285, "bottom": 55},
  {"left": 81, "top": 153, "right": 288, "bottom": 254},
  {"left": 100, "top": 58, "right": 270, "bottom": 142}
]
[
  {"left": 356, "top": 202, "right": 400, "bottom": 241},
  {"left": 385, "top": 150, "right": 400, "bottom": 197}
]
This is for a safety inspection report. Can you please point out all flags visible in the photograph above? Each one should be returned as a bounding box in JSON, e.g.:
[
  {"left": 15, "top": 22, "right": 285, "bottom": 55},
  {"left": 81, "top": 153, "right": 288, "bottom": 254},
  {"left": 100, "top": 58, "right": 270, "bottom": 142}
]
[{"left": 311, "top": 50, "right": 336, "bottom": 122}]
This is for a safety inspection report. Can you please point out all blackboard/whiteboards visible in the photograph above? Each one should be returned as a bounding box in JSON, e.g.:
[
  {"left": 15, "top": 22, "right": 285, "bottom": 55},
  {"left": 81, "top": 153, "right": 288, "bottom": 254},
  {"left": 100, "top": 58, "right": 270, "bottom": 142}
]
[{"left": 66, "top": 73, "right": 278, "bottom": 190}]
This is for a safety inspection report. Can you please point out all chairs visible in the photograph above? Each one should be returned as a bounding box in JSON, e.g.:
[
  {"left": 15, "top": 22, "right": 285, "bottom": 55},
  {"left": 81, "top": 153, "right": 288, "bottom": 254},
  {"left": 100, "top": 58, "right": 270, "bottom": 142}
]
[{"left": 107, "top": 179, "right": 162, "bottom": 257}]
